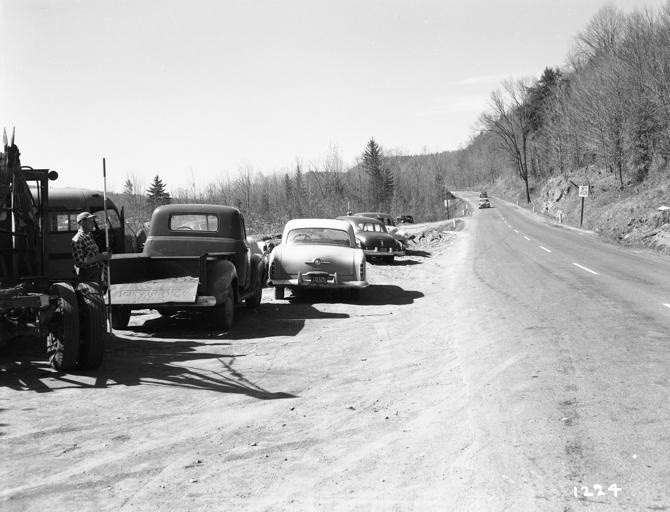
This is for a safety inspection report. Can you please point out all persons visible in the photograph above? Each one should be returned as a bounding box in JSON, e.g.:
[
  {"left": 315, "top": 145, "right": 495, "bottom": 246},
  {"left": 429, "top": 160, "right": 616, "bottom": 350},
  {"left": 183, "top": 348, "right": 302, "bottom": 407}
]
[{"left": 70, "top": 211, "right": 112, "bottom": 285}]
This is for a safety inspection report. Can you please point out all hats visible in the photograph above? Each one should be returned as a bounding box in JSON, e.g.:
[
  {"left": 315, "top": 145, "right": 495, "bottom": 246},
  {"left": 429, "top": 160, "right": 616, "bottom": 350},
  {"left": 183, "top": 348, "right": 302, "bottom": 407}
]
[{"left": 76, "top": 212, "right": 95, "bottom": 223}]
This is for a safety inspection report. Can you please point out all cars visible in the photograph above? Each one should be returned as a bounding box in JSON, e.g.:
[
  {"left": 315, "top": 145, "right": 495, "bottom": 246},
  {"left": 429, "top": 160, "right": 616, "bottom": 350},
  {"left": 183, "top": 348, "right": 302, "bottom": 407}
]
[
  {"left": 0, "top": 124, "right": 415, "bottom": 377},
  {"left": 480, "top": 191, "right": 488, "bottom": 199},
  {"left": 477, "top": 198, "right": 491, "bottom": 209}
]
